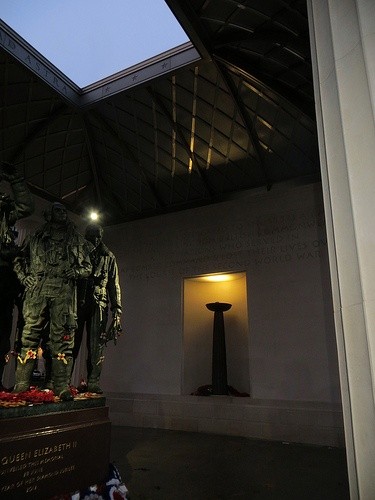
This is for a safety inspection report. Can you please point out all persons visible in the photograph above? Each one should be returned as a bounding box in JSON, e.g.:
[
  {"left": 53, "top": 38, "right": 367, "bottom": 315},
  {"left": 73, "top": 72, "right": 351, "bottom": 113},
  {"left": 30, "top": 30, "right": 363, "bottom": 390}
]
[
  {"left": 1, "top": 162, "right": 34, "bottom": 392},
  {"left": 13, "top": 203, "right": 91, "bottom": 402},
  {"left": 70, "top": 220, "right": 122, "bottom": 394}
]
